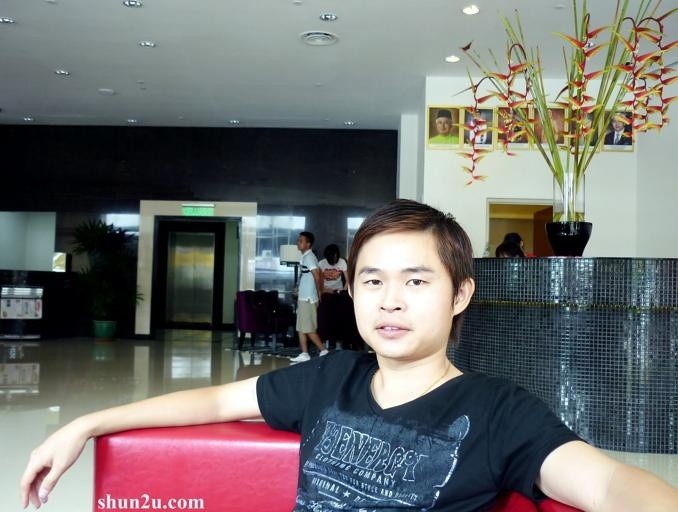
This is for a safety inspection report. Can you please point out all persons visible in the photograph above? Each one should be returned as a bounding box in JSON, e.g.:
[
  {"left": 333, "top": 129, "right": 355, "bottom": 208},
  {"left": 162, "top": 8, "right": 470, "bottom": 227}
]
[
  {"left": 470, "top": 110, "right": 488, "bottom": 145},
  {"left": 289, "top": 230, "right": 330, "bottom": 363},
  {"left": 316, "top": 242, "right": 349, "bottom": 350},
  {"left": 17, "top": 197, "right": 678, "bottom": 511},
  {"left": 430, "top": 109, "right": 460, "bottom": 145},
  {"left": 604, "top": 112, "right": 631, "bottom": 145},
  {"left": 494, "top": 231, "right": 526, "bottom": 257}
]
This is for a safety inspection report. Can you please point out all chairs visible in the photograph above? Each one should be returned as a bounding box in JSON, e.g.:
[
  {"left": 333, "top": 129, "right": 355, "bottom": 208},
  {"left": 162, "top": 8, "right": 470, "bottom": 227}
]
[{"left": 233, "top": 288, "right": 291, "bottom": 348}]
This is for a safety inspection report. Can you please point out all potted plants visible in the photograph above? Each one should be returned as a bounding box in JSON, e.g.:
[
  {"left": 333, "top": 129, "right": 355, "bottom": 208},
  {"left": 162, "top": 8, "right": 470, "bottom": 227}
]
[
  {"left": 449, "top": 1, "right": 677, "bottom": 256},
  {"left": 69, "top": 221, "right": 142, "bottom": 343}
]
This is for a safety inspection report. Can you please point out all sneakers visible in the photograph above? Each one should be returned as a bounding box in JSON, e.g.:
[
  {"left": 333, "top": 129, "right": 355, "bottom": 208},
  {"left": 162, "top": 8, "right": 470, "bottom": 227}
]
[
  {"left": 290, "top": 353, "right": 311, "bottom": 362},
  {"left": 319, "top": 350, "right": 328, "bottom": 356}
]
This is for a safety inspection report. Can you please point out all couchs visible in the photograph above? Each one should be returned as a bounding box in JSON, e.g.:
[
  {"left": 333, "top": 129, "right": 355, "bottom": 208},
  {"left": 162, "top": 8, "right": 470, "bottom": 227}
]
[{"left": 92, "top": 416, "right": 580, "bottom": 510}]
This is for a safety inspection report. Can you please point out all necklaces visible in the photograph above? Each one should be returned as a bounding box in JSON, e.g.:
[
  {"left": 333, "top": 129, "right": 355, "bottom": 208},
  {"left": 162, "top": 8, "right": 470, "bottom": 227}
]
[{"left": 368, "top": 359, "right": 452, "bottom": 401}]
